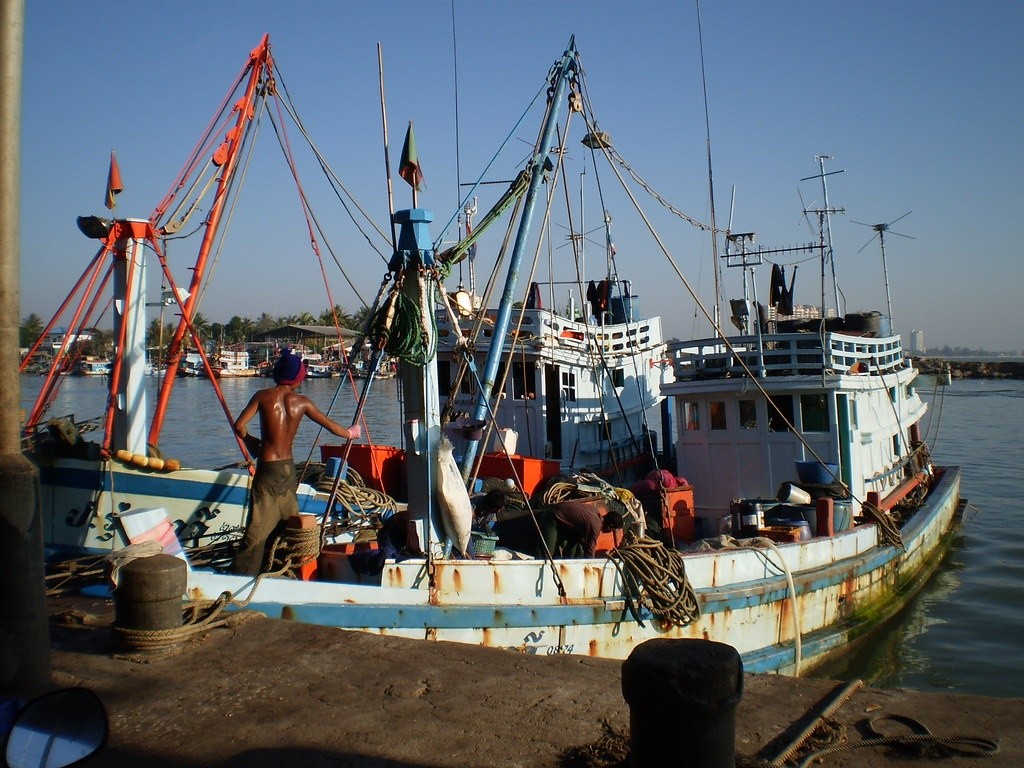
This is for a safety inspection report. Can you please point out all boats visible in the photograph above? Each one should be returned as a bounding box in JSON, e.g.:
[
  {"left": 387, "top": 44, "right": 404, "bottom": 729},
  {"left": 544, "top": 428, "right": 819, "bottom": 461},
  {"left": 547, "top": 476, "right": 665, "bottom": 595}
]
[
  {"left": 35, "top": 348, "right": 393, "bottom": 382},
  {"left": 12, "top": 32, "right": 967, "bottom": 681}
]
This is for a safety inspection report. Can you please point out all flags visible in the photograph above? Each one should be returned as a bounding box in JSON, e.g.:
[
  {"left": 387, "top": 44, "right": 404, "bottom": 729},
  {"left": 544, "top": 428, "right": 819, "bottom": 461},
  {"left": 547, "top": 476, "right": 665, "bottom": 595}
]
[
  {"left": 399, "top": 123, "right": 429, "bottom": 194},
  {"left": 105, "top": 155, "right": 123, "bottom": 209}
]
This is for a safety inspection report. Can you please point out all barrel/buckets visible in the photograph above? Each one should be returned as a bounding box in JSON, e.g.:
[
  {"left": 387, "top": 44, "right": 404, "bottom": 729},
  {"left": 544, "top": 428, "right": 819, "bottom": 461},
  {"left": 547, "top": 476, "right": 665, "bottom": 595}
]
[{"left": 729, "top": 498, "right": 852, "bottom": 544}]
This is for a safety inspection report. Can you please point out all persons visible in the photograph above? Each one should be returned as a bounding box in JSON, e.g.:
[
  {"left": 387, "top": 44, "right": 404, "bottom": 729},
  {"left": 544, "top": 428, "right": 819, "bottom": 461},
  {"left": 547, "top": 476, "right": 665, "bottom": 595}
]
[
  {"left": 540, "top": 502, "right": 622, "bottom": 561},
  {"left": 471, "top": 488, "right": 507, "bottom": 537},
  {"left": 231, "top": 350, "right": 362, "bottom": 578}
]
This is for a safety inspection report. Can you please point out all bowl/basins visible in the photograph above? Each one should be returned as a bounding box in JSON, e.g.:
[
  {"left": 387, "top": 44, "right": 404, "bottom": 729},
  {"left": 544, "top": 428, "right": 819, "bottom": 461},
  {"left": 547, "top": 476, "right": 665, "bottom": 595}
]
[{"left": 796, "top": 460, "right": 838, "bottom": 485}]
